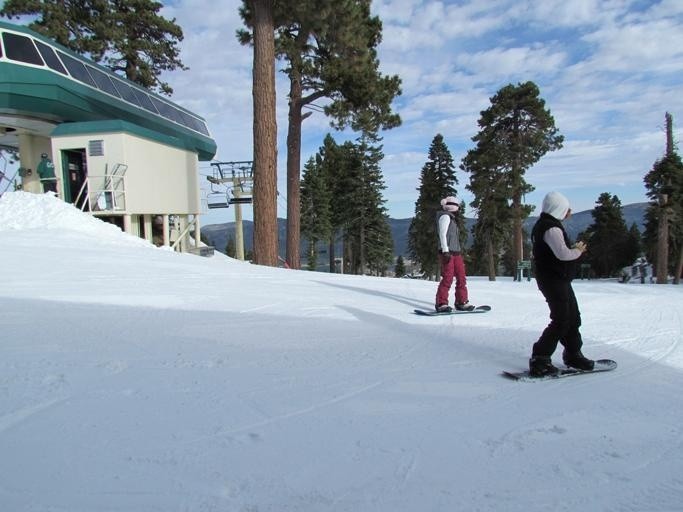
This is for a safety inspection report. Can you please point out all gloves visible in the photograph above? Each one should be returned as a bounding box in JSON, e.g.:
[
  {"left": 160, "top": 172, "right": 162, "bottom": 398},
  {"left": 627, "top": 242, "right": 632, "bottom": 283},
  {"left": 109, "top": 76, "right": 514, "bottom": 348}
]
[
  {"left": 571, "top": 241, "right": 586, "bottom": 253},
  {"left": 442, "top": 252, "right": 450, "bottom": 265}
]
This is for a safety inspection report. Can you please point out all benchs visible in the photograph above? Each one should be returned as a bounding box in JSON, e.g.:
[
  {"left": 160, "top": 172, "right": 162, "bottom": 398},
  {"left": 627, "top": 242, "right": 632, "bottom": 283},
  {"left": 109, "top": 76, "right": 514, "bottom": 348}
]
[
  {"left": 208, "top": 202, "right": 229, "bottom": 208},
  {"left": 227, "top": 198, "right": 252, "bottom": 203}
]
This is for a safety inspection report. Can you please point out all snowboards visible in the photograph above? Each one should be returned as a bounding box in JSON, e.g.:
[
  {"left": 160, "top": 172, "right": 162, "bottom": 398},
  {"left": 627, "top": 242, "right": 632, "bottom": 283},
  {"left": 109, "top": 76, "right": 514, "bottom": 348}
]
[
  {"left": 413, "top": 305, "right": 491, "bottom": 317},
  {"left": 502, "top": 359, "right": 618, "bottom": 382}
]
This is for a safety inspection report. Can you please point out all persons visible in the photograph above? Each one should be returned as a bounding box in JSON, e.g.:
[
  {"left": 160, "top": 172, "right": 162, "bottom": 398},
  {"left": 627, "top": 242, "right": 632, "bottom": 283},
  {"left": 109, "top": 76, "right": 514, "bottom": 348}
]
[
  {"left": 434, "top": 196, "right": 475, "bottom": 313},
  {"left": 36, "top": 152, "right": 57, "bottom": 197},
  {"left": 526, "top": 190, "right": 598, "bottom": 376}
]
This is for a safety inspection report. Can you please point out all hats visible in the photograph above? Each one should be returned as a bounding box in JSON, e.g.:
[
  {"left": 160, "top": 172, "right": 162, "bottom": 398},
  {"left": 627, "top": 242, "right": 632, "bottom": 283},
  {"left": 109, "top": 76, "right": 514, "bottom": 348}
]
[{"left": 440, "top": 196, "right": 460, "bottom": 205}]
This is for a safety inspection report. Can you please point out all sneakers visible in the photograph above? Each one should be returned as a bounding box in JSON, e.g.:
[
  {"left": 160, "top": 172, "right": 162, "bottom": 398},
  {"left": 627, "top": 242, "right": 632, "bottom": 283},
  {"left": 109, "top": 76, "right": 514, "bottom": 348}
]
[
  {"left": 436, "top": 303, "right": 475, "bottom": 313},
  {"left": 529, "top": 354, "right": 594, "bottom": 376}
]
